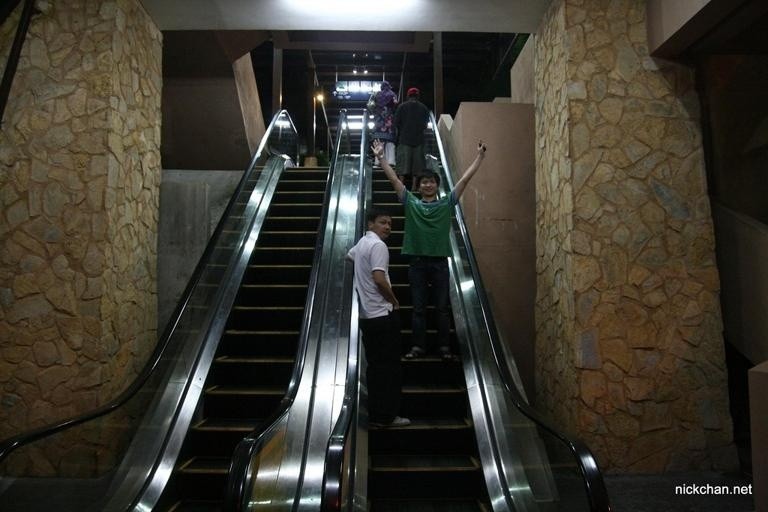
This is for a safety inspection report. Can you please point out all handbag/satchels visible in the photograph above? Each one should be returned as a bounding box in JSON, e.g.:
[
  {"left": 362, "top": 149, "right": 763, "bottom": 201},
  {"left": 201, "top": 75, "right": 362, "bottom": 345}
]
[{"left": 366, "top": 94, "right": 376, "bottom": 113}]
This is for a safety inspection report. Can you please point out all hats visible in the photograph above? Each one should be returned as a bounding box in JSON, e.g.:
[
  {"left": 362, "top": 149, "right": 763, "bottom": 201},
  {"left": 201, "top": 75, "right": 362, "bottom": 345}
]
[{"left": 406, "top": 87, "right": 420, "bottom": 97}]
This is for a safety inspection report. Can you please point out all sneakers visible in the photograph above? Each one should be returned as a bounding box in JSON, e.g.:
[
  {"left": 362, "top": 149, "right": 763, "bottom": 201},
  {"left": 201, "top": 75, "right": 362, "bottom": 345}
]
[
  {"left": 404, "top": 347, "right": 453, "bottom": 359},
  {"left": 371, "top": 414, "right": 411, "bottom": 427}
]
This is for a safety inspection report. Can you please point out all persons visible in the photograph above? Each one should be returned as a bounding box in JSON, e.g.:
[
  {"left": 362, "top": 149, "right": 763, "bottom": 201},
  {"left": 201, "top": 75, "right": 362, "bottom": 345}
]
[
  {"left": 369, "top": 135, "right": 487, "bottom": 364},
  {"left": 369, "top": 80, "right": 398, "bottom": 171},
  {"left": 344, "top": 209, "right": 411, "bottom": 429},
  {"left": 390, "top": 86, "right": 429, "bottom": 194}
]
